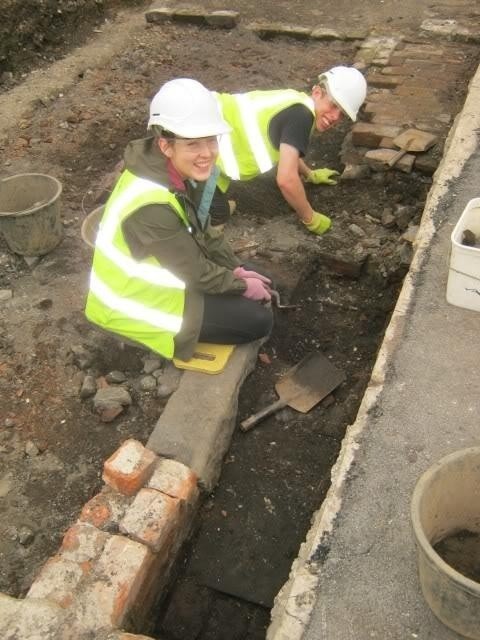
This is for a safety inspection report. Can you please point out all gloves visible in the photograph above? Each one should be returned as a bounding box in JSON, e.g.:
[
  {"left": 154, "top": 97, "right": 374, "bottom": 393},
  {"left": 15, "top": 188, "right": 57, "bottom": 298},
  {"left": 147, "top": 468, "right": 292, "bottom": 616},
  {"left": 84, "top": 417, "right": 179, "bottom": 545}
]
[
  {"left": 303, "top": 168, "right": 340, "bottom": 186},
  {"left": 302, "top": 211, "right": 332, "bottom": 235},
  {"left": 232, "top": 266, "right": 272, "bottom": 301}
]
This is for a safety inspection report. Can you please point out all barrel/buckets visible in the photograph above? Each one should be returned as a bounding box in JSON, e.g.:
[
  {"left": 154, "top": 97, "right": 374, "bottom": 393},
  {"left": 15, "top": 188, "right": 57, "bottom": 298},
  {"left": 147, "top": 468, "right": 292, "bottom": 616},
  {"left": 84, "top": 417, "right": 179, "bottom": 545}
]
[
  {"left": 0, "top": 173, "right": 62, "bottom": 257},
  {"left": 81, "top": 186, "right": 110, "bottom": 248},
  {"left": 410, "top": 446, "right": 480, "bottom": 640}
]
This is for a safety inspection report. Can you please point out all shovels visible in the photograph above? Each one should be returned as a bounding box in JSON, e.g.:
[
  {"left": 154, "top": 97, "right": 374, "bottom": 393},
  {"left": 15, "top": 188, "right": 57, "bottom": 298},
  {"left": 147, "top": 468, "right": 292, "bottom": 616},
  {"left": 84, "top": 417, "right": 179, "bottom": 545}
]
[
  {"left": 385, "top": 128, "right": 438, "bottom": 171},
  {"left": 238, "top": 349, "right": 344, "bottom": 433}
]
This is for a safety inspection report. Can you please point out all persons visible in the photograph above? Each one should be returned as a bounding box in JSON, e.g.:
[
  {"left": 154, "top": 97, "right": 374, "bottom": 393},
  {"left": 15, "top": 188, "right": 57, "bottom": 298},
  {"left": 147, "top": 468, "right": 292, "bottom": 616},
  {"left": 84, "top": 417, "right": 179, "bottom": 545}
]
[
  {"left": 91, "top": 63, "right": 370, "bottom": 236},
  {"left": 83, "top": 77, "right": 275, "bottom": 375}
]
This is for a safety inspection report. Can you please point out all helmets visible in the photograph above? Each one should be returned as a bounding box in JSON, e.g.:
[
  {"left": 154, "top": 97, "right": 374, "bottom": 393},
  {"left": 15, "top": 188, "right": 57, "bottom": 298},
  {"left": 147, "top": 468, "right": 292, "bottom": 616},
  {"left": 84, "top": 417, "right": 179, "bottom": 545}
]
[
  {"left": 319, "top": 66, "right": 367, "bottom": 123},
  {"left": 147, "top": 78, "right": 233, "bottom": 138}
]
